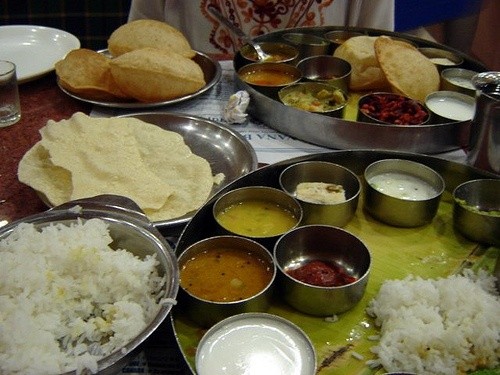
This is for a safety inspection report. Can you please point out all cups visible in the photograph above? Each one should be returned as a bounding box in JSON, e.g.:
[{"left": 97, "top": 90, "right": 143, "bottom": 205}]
[{"left": 0, "top": 61, "right": 22, "bottom": 128}]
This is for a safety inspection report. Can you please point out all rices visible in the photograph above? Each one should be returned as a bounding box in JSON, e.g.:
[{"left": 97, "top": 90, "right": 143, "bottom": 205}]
[
  {"left": 0, "top": 217, "right": 178, "bottom": 375},
  {"left": 326, "top": 265, "right": 500, "bottom": 375}
]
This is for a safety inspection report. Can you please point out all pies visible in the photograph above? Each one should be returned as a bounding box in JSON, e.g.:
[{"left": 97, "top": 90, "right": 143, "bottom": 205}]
[
  {"left": 54, "top": 48, "right": 131, "bottom": 99},
  {"left": 331, "top": 34, "right": 390, "bottom": 91},
  {"left": 108, "top": 48, "right": 205, "bottom": 103},
  {"left": 374, "top": 36, "right": 440, "bottom": 104},
  {"left": 107, "top": 19, "right": 197, "bottom": 58},
  {"left": 15, "top": 112, "right": 213, "bottom": 224}
]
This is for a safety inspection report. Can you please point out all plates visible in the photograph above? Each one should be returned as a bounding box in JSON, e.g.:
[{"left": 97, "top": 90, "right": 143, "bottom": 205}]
[
  {"left": 1, "top": 194, "right": 179, "bottom": 374},
  {"left": 34, "top": 112, "right": 259, "bottom": 228},
  {"left": 57, "top": 47, "right": 222, "bottom": 109},
  {"left": 0, "top": 25, "right": 81, "bottom": 84}
]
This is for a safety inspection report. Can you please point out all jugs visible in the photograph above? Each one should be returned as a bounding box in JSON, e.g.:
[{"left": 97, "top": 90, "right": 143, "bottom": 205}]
[{"left": 470, "top": 72, "right": 500, "bottom": 173}]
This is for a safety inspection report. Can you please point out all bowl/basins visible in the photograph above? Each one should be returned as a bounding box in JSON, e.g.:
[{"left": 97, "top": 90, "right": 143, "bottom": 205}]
[
  {"left": 212, "top": 185, "right": 303, "bottom": 249},
  {"left": 193, "top": 313, "right": 318, "bottom": 375},
  {"left": 178, "top": 235, "right": 276, "bottom": 324},
  {"left": 365, "top": 158, "right": 500, "bottom": 247},
  {"left": 238, "top": 29, "right": 482, "bottom": 124},
  {"left": 279, "top": 161, "right": 362, "bottom": 228},
  {"left": 273, "top": 224, "right": 372, "bottom": 316}
]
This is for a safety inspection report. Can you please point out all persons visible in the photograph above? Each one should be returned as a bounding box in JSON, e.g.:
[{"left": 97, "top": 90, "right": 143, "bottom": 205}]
[{"left": 127, "top": 0, "right": 395, "bottom": 55}]
